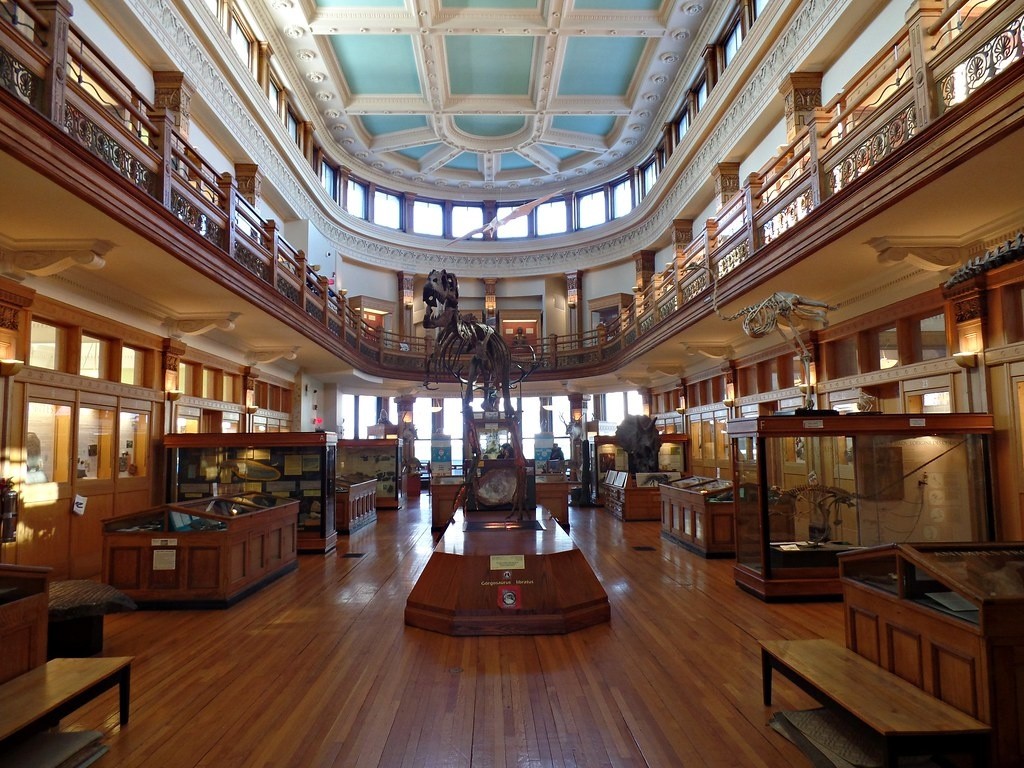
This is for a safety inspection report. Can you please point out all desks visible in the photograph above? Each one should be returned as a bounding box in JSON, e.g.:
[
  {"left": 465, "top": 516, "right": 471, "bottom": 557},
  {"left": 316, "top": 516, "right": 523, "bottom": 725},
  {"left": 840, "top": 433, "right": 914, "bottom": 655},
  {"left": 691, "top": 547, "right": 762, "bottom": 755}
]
[{"left": 45, "top": 577, "right": 136, "bottom": 662}]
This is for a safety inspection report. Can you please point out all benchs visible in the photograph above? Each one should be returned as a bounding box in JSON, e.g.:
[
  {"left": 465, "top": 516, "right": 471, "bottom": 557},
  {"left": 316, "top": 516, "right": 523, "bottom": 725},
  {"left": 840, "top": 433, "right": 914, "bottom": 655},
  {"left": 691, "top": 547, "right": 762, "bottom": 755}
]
[
  {"left": 0, "top": 656, "right": 135, "bottom": 768},
  {"left": 757, "top": 637, "right": 992, "bottom": 768}
]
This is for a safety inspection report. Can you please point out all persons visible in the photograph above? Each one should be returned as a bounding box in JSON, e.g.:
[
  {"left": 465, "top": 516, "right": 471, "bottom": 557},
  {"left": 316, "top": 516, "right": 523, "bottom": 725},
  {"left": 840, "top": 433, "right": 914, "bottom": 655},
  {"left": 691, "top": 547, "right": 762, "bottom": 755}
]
[
  {"left": 549, "top": 444, "right": 564, "bottom": 474},
  {"left": 497, "top": 443, "right": 514, "bottom": 459},
  {"left": 513, "top": 327, "right": 526, "bottom": 354}
]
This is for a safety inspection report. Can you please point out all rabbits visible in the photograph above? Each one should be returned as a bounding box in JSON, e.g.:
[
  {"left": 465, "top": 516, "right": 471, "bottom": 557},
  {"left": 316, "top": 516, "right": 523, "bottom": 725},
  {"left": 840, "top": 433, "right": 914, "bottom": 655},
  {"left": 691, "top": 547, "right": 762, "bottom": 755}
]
[{"left": 378, "top": 408, "right": 389, "bottom": 425}]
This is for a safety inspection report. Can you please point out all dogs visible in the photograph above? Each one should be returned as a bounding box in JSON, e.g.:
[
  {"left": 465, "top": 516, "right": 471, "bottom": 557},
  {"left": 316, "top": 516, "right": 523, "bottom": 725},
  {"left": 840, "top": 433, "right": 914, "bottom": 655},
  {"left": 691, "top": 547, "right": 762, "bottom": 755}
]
[{"left": 403, "top": 456, "right": 425, "bottom": 471}]
